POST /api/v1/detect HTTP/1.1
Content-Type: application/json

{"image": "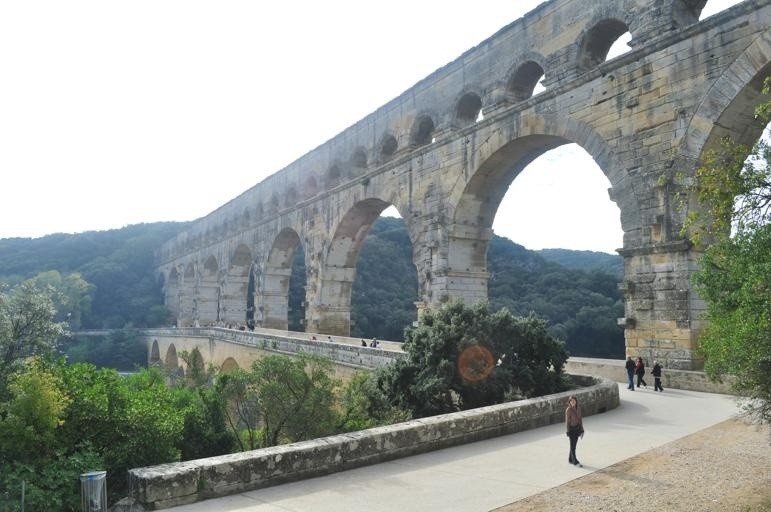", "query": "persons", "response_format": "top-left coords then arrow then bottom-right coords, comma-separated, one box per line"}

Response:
635,356 -> 647,389
565,396 -> 584,468
327,336 -> 336,342
625,356 -> 636,391
651,361 -> 664,392
171,316 -> 256,331
361,337 -> 382,348
310,336 -> 317,340
